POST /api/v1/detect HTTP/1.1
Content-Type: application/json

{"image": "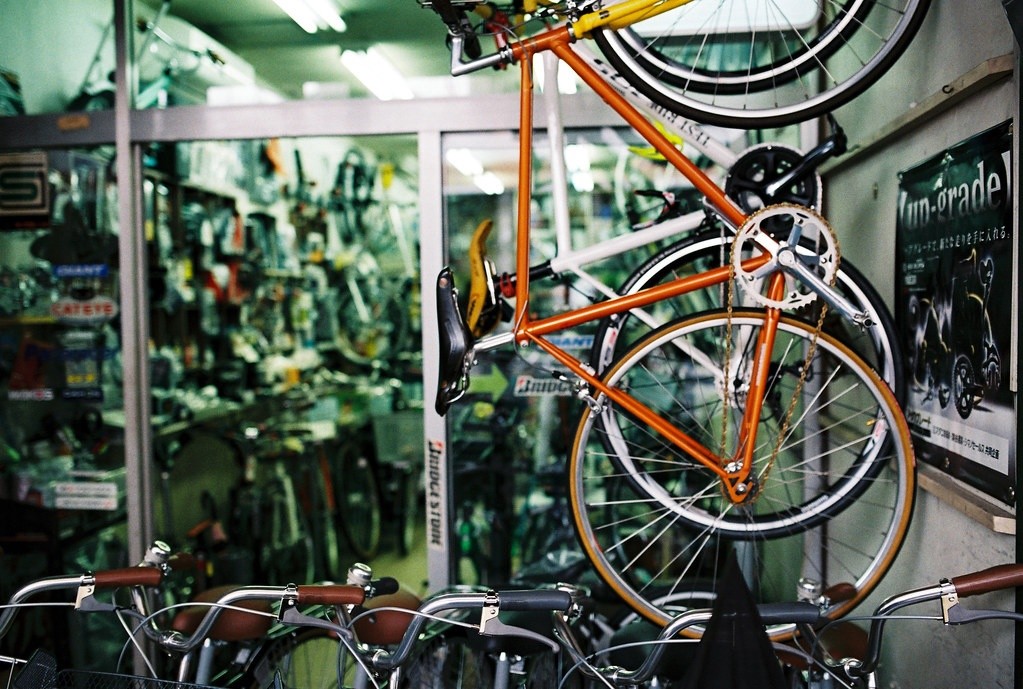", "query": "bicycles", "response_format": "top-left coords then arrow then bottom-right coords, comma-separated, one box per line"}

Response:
0,0 -> 1022,689
